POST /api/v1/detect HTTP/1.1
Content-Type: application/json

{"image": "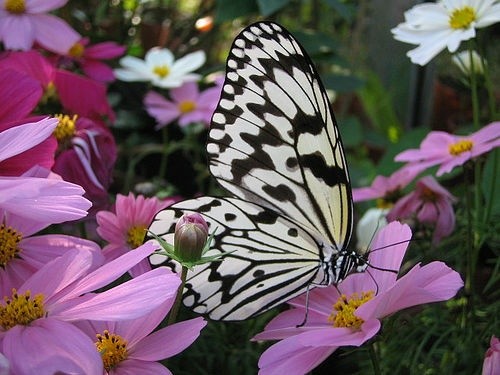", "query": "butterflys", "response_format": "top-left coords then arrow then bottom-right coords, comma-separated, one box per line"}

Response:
142,22 -> 420,328
